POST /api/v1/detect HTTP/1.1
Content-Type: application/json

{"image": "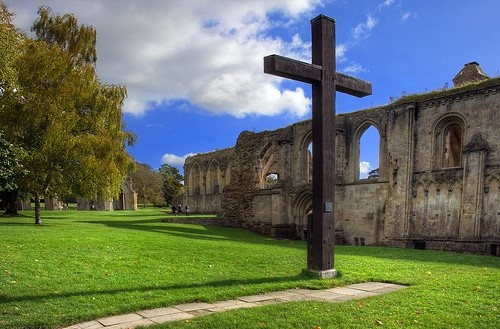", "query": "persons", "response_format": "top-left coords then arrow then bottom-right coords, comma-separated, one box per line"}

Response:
178,203 -> 184,216
89,205 -> 97,210
185,204 -> 190,216
172,203 -> 177,216
